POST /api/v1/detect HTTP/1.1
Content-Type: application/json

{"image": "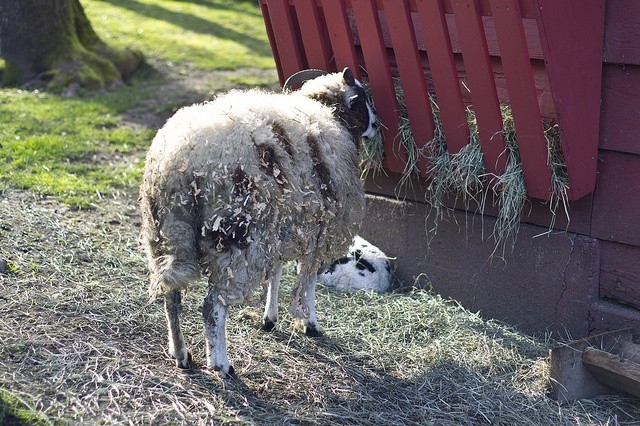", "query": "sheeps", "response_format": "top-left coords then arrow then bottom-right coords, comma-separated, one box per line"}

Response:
139,66 -> 378,378
294,234 -> 397,296
